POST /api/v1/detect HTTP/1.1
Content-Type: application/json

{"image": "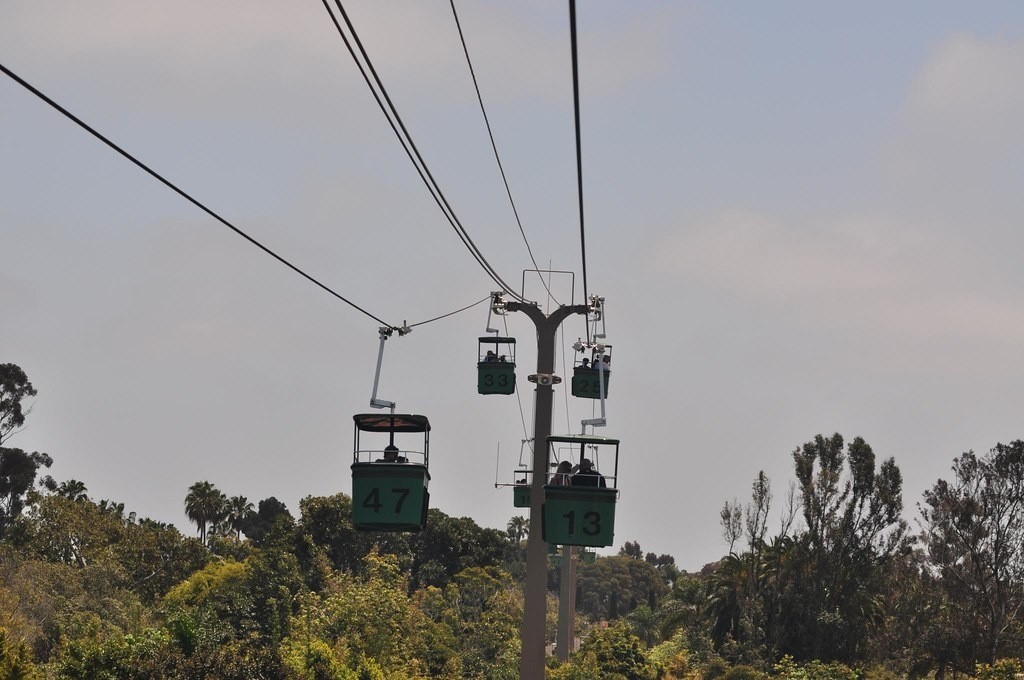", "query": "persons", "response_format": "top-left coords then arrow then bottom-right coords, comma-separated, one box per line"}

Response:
578,355 -> 611,369
485,350 -> 506,362
375,445 -> 408,463
549,459 -> 606,488
